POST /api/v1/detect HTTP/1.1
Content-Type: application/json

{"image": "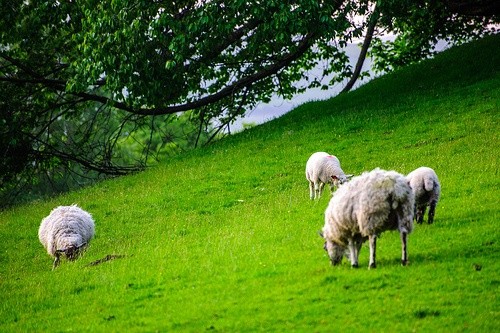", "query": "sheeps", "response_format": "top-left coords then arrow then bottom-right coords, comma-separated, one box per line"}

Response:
404,166 -> 440,225
305,152 -> 354,200
319,168 -> 415,271
38,203 -> 96,271
